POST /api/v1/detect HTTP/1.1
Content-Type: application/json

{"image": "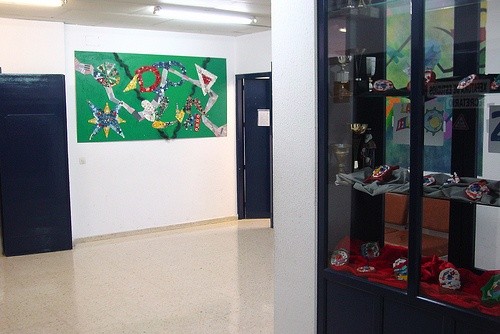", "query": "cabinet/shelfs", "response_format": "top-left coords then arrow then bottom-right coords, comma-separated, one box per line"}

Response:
315,0 -> 500,334
